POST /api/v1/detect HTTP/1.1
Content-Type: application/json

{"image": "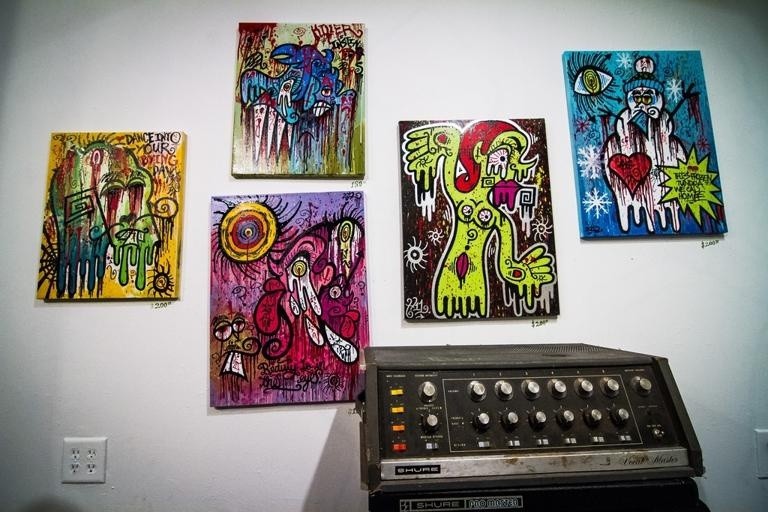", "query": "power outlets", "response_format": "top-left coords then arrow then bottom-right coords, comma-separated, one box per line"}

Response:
62,437 -> 108,484
